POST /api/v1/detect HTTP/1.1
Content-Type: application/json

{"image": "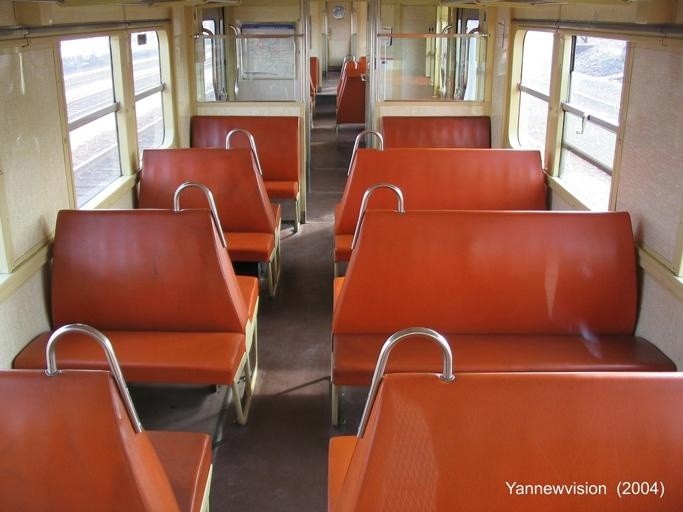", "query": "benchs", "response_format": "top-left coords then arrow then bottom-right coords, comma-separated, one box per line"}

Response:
0,56 -> 683,512
